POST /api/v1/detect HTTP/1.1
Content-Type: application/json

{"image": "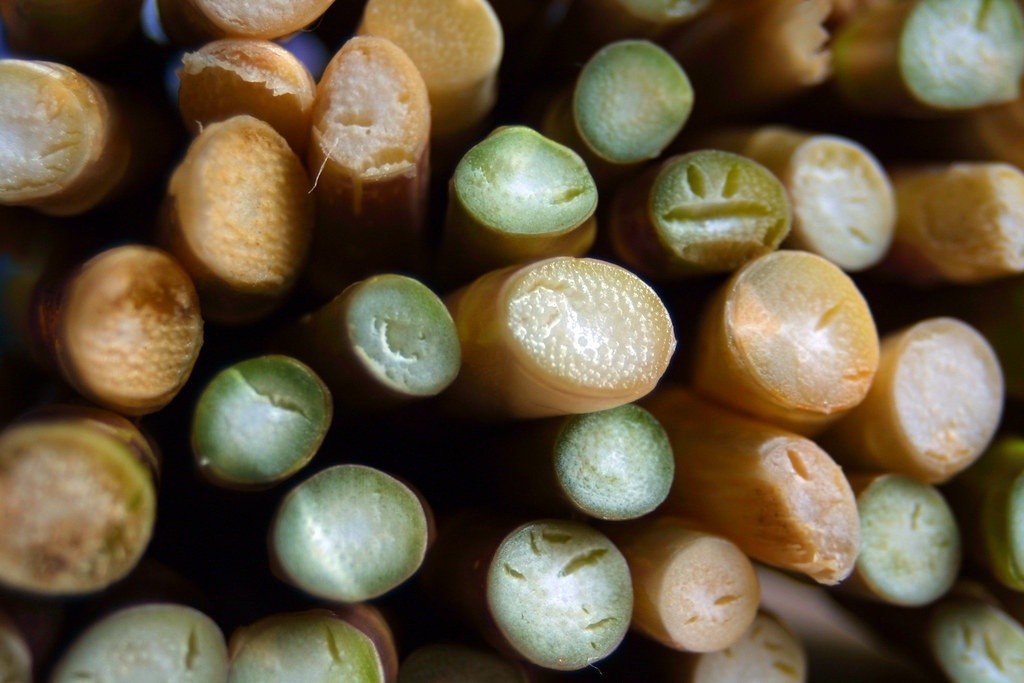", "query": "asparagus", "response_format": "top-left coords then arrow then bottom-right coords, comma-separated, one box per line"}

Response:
0,0 -> 1024,683
